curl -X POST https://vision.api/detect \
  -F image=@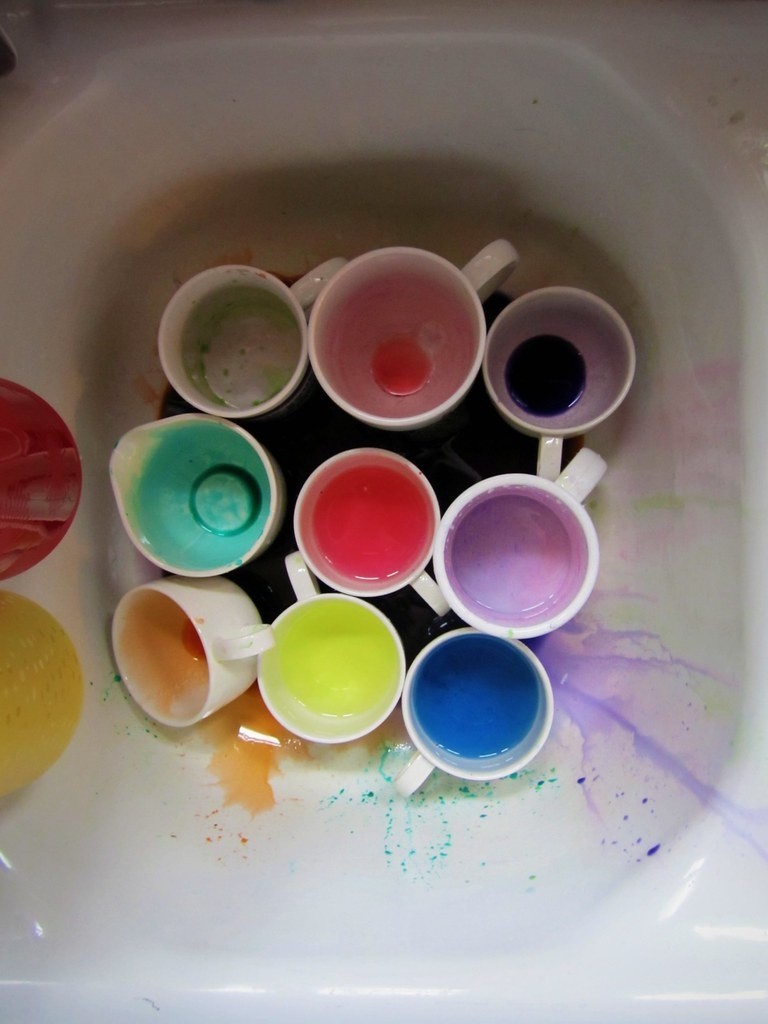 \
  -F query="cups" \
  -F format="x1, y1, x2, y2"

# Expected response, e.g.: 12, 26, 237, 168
433, 447, 606, 640
482, 287, 635, 480
0, 588, 85, 800
113, 577, 273, 726
397, 627, 554, 797
158, 257, 347, 418
109, 414, 286, 578
256, 552, 406, 743
308, 239, 520, 430
293, 447, 451, 618
0, 376, 83, 584
